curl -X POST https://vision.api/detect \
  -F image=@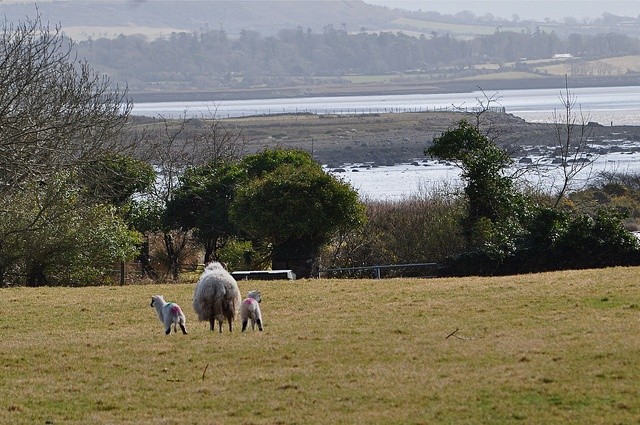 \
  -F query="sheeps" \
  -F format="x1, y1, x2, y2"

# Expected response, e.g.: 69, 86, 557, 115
149, 294, 189, 336
240, 290, 264, 333
192, 259, 241, 334
